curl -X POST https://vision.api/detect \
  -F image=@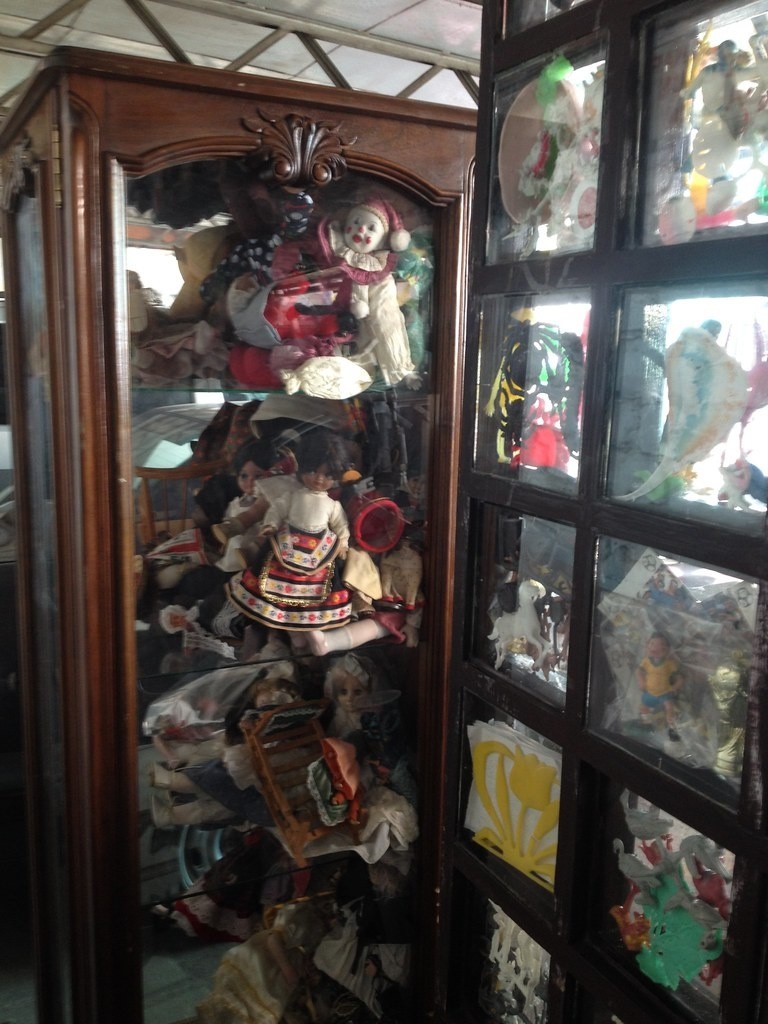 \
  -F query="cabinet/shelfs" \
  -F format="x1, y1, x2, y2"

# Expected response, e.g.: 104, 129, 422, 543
0, 44, 479, 1023
434, 0, 768, 1024
